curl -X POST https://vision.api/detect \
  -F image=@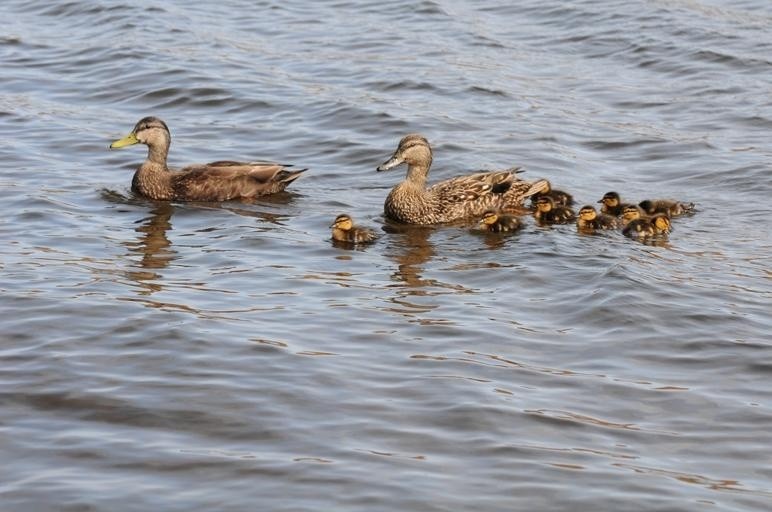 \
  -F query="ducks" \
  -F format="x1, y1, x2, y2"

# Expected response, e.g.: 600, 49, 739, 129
377, 132, 700, 239
328, 212, 386, 244
106, 113, 309, 202
